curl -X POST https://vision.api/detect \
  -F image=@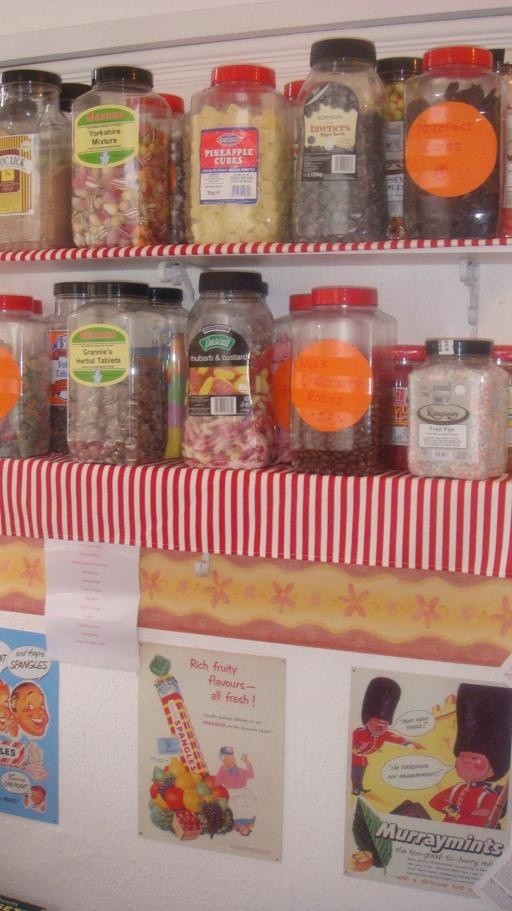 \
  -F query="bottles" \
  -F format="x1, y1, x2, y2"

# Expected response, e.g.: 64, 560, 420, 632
146, 285, 192, 459
180, 269, 276, 469
0, 294, 52, 457
405, 338, 510, 481
290, 36, 388, 242
71, 65, 172, 249
378, 55, 425, 239
45, 281, 88, 453
158, 93, 186, 243
65, 281, 170, 466
273, 293, 312, 463
189, 64, 295, 243
288, 284, 398, 475
0, 68, 68, 251
402, 45, 509, 239
392, 344, 428, 470
